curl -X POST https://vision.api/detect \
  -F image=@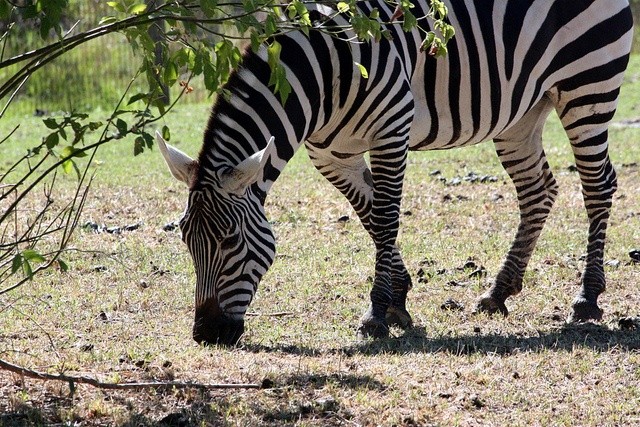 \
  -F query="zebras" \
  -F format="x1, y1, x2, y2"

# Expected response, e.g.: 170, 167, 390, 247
155, 0, 634, 349
